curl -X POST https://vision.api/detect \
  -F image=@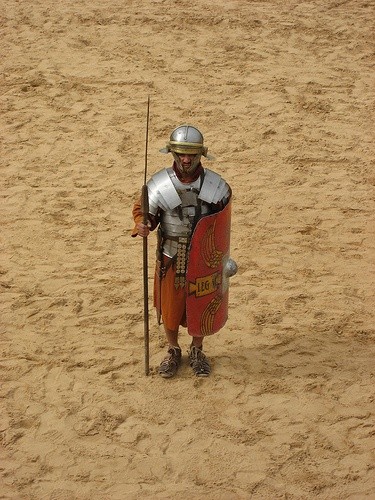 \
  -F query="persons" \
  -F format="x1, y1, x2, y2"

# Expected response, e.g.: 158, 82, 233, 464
130, 122, 233, 377
